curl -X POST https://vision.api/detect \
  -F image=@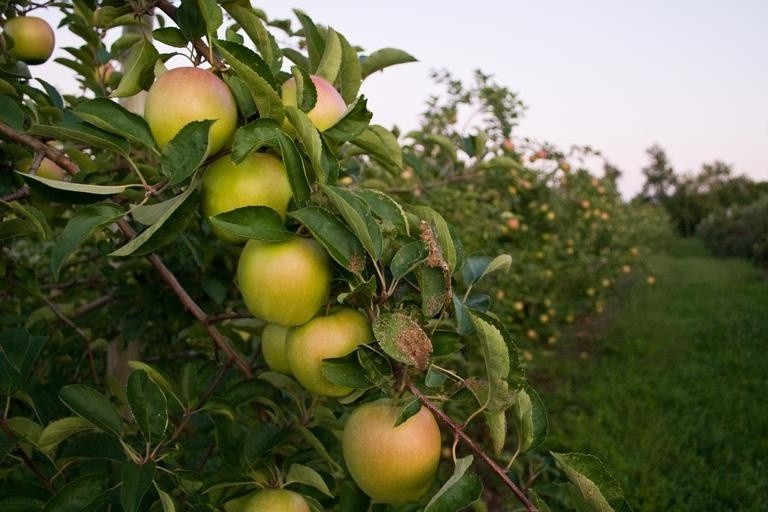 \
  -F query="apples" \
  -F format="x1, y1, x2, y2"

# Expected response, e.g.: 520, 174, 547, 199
246, 489, 308, 511
279, 76, 347, 132
13, 159, 66, 184
343, 399, 440, 503
201, 151, 297, 247
337, 138, 661, 360
146, 67, 237, 157
2, 17, 54, 65
91, 64, 118, 83
236, 233, 370, 397
